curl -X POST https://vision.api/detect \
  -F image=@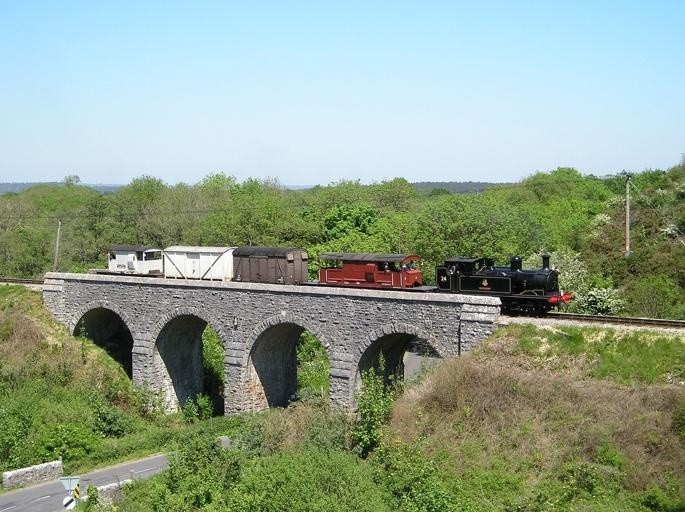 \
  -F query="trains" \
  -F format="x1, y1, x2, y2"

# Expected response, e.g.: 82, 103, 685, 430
108, 243, 573, 318
